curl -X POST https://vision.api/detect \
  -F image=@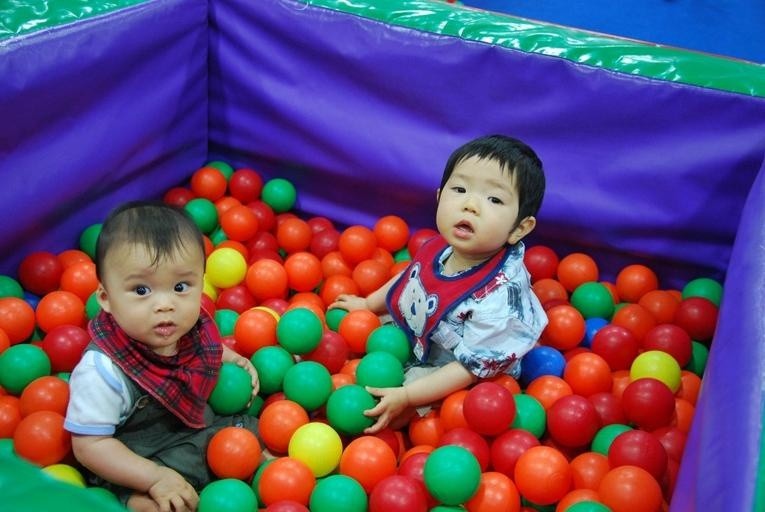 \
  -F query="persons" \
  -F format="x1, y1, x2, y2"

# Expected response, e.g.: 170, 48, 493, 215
62, 198, 266, 511
328, 137, 550, 434
